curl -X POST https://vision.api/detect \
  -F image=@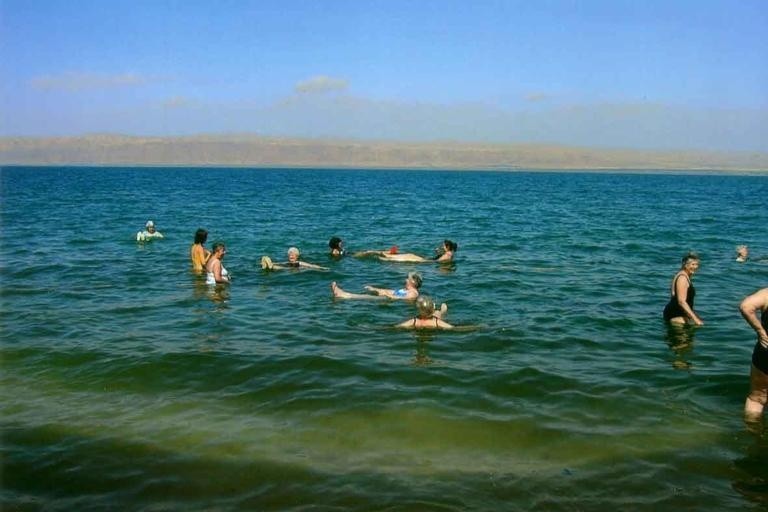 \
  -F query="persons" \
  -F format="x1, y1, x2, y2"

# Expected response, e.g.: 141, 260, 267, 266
190, 227, 211, 269
330, 272, 423, 299
379, 239, 458, 263
328, 237, 398, 259
663, 254, 704, 325
205, 241, 231, 285
260, 247, 330, 272
382, 295, 474, 331
739, 287, 768, 420
136, 220, 163, 241
735, 244, 749, 263
